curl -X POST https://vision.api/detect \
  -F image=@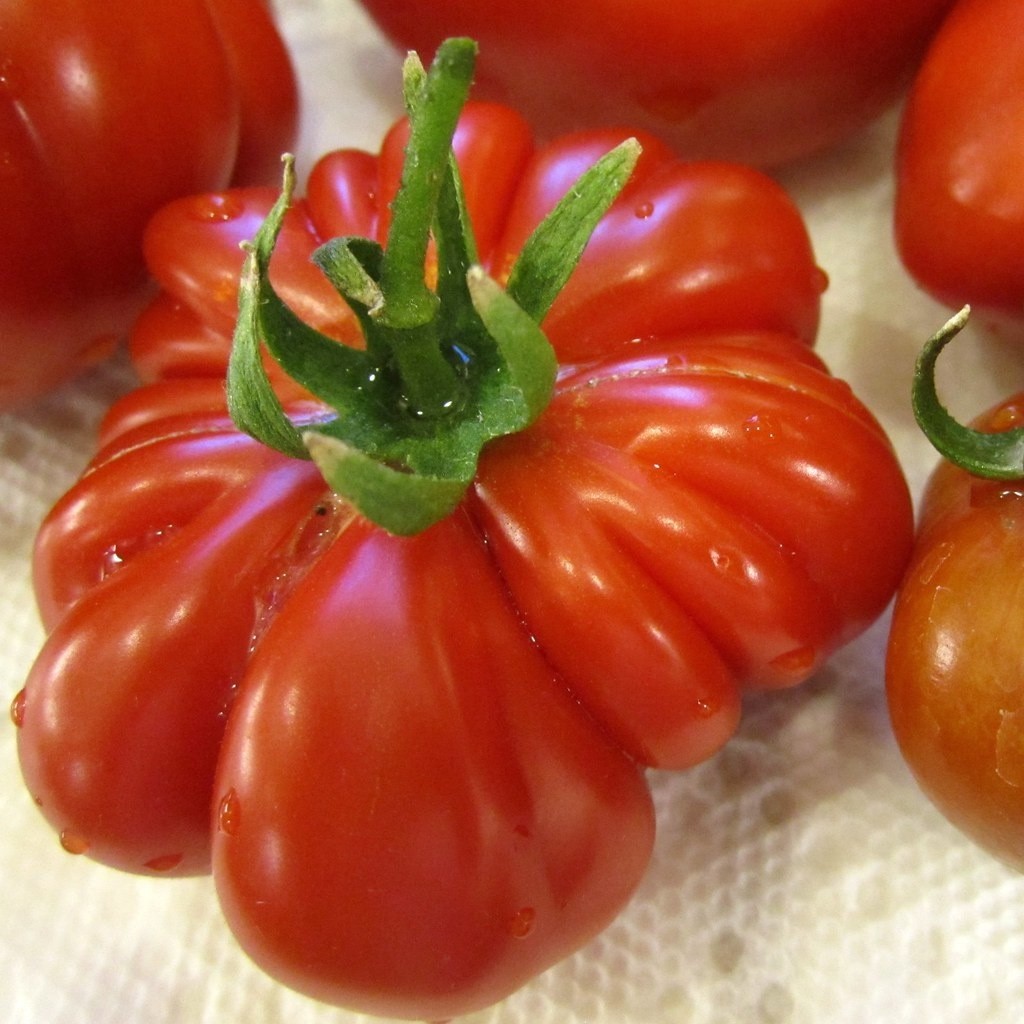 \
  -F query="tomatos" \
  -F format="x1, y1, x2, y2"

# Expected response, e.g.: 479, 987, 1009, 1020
0, 0, 1024, 1024
